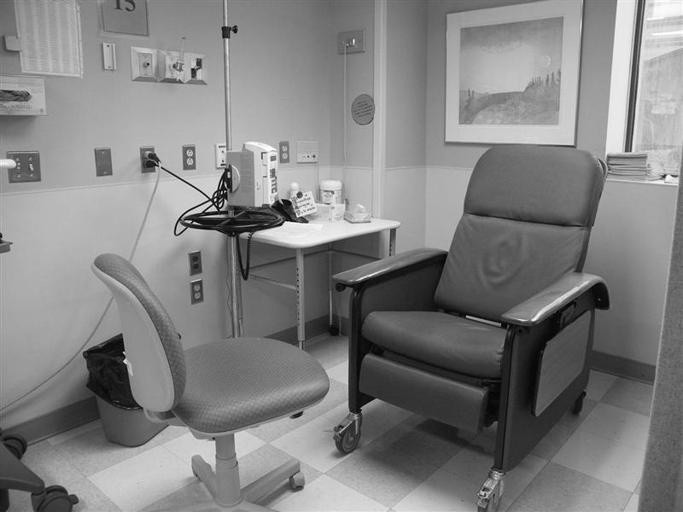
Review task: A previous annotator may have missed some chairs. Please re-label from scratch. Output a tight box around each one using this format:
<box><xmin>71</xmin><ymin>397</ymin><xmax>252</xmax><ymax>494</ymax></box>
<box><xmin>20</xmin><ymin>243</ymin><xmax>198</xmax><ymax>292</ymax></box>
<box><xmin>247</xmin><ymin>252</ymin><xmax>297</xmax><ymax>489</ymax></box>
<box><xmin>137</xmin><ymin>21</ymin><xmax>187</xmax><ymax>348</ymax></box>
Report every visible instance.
<box><xmin>91</xmin><ymin>253</ymin><xmax>330</xmax><ymax>512</ymax></box>
<box><xmin>329</xmin><ymin>147</ymin><xmax>611</xmax><ymax>512</ymax></box>
<box><xmin>0</xmin><ymin>426</ymin><xmax>79</xmax><ymax>511</ymax></box>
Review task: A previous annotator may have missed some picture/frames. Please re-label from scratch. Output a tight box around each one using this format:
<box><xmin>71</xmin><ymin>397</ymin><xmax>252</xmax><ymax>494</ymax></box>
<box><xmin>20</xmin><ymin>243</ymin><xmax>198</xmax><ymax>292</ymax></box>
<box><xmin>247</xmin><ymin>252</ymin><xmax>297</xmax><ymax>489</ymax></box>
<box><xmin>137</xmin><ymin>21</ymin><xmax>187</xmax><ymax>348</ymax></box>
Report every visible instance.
<box><xmin>444</xmin><ymin>0</ymin><xmax>585</xmax><ymax>149</ymax></box>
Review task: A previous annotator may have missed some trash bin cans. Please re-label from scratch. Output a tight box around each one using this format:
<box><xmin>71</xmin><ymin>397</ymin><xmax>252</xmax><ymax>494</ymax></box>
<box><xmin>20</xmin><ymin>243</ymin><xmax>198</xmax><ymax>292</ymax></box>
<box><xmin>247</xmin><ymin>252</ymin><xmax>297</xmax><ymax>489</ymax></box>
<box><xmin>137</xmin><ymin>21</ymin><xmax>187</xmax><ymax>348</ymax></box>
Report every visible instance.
<box><xmin>82</xmin><ymin>332</ymin><xmax>182</xmax><ymax>447</ymax></box>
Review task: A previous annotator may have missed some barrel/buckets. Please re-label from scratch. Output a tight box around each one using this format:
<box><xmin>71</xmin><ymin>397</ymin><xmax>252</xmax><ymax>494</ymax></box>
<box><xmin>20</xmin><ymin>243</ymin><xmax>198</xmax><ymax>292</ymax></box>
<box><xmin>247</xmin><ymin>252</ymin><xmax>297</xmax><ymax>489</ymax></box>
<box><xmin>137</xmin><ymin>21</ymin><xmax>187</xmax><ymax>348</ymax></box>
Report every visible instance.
<box><xmin>320</xmin><ymin>180</ymin><xmax>342</xmax><ymax>205</ymax></box>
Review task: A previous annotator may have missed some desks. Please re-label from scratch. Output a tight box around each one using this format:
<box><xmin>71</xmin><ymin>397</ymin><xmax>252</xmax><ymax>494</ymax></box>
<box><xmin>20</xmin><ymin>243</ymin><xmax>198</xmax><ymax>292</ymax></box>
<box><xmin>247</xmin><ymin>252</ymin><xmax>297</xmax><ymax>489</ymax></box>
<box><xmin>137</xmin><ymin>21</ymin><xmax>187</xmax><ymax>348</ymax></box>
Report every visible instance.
<box><xmin>227</xmin><ymin>204</ymin><xmax>400</xmax><ymax>351</ymax></box>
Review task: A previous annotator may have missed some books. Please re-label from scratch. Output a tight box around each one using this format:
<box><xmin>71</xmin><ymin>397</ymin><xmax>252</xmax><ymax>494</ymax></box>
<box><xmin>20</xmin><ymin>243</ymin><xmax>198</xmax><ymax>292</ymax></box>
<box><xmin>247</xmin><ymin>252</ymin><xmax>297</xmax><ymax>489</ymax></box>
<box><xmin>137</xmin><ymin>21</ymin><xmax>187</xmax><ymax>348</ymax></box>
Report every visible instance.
<box><xmin>606</xmin><ymin>151</ymin><xmax>649</xmax><ymax>181</ymax></box>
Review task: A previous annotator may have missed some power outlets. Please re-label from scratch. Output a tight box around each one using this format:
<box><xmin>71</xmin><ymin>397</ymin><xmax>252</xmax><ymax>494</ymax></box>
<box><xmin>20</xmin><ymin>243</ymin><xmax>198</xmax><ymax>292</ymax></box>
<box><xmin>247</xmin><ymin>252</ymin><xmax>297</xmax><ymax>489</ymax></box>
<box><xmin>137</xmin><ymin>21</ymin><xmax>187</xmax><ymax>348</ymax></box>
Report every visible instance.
<box><xmin>184</xmin><ymin>145</ymin><xmax>196</xmax><ymax>170</ymax></box>
<box><xmin>141</xmin><ymin>146</ymin><xmax>156</xmax><ymax>172</ymax></box>
